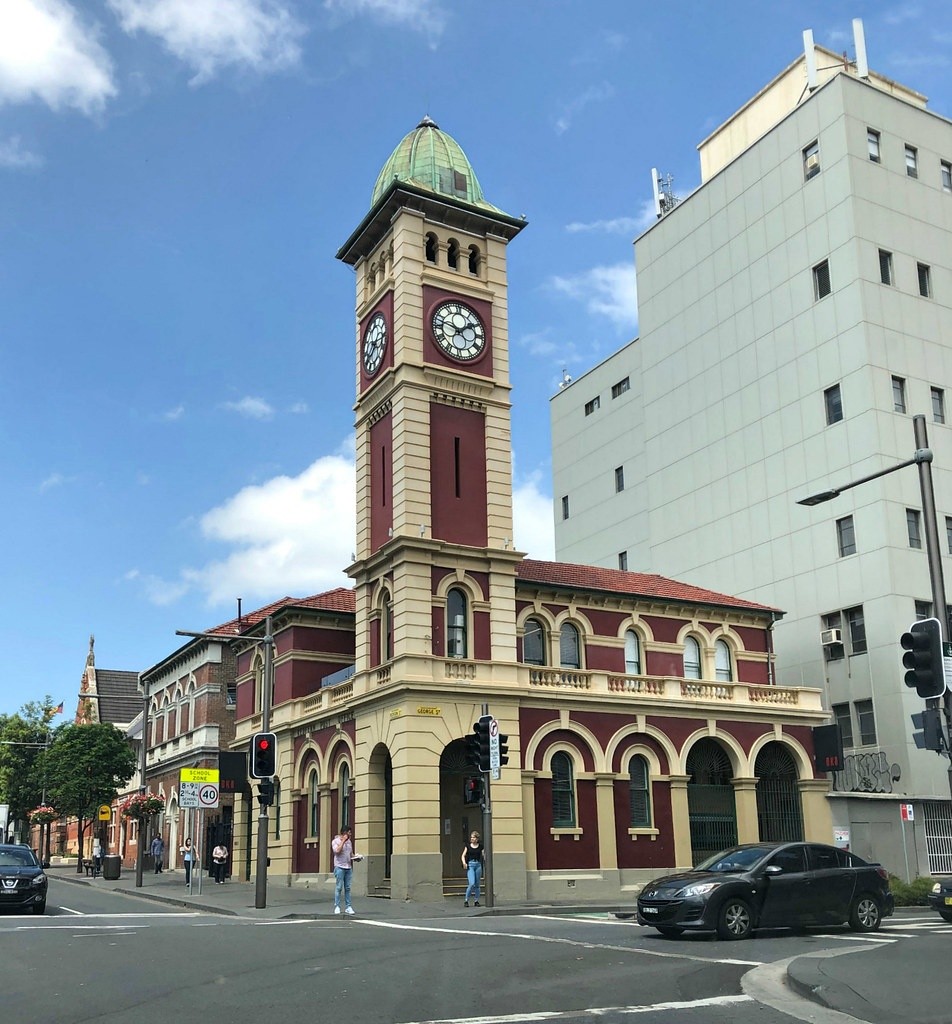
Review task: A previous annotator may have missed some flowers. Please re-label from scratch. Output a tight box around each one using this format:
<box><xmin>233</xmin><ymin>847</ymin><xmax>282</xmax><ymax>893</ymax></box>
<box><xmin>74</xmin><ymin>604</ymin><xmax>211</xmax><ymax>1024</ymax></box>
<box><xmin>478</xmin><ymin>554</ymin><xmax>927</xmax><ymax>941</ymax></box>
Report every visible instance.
<box><xmin>29</xmin><ymin>806</ymin><xmax>55</xmax><ymax>824</ymax></box>
<box><xmin>119</xmin><ymin>791</ymin><xmax>164</xmax><ymax>818</ymax></box>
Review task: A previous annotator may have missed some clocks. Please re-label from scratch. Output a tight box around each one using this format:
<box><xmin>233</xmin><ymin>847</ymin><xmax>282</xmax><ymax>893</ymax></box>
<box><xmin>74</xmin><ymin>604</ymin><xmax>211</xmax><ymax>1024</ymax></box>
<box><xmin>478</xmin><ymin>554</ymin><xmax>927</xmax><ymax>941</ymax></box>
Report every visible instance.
<box><xmin>428</xmin><ymin>296</ymin><xmax>489</xmax><ymax>365</ymax></box>
<box><xmin>359</xmin><ymin>311</ymin><xmax>387</xmax><ymax>379</ymax></box>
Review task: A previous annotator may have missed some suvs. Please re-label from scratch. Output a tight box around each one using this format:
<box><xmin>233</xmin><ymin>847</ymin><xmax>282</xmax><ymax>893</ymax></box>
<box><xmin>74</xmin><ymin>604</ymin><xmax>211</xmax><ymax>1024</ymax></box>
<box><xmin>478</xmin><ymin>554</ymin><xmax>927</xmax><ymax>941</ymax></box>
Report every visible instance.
<box><xmin>0</xmin><ymin>842</ymin><xmax>51</xmax><ymax>915</ymax></box>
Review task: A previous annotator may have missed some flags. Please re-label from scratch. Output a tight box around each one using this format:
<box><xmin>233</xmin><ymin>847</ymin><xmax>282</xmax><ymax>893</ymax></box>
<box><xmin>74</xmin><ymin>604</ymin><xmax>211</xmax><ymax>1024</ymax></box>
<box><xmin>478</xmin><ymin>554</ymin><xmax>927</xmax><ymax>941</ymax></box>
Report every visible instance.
<box><xmin>51</xmin><ymin>702</ymin><xmax>64</xmax><ymax>713</ymax></box>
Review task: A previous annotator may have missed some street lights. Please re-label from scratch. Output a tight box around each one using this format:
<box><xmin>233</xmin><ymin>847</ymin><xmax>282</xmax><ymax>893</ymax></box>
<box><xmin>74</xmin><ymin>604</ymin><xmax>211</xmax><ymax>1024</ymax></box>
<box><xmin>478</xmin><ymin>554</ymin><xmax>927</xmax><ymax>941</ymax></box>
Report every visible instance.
<box><xmin>2</xmin><ymin>733</ymin><xmax>48</xmax><ymax>864</ymax></box>
<box><xmin>175</xmin><ymin>616</ymin><xmax>274</xmax><ymax>908</ymax></box>
<box><xmin>795</xmin><ymin>414</ymin><xmax>952</xmax><ymax>798</ymax></box>
<box><xmin>78</xmin><ymin>681</ymin><xmax>151</xmax><ymax>887</ymax></box>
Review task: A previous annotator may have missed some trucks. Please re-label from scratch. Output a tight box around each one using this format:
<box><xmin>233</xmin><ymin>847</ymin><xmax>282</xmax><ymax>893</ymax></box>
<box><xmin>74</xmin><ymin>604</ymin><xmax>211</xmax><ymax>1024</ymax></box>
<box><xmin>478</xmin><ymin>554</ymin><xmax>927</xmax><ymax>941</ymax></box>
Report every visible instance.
<box><xmin>0</xmin><ymin>804</ymin><xmax>8</xmax><ymax>843</ymax></box>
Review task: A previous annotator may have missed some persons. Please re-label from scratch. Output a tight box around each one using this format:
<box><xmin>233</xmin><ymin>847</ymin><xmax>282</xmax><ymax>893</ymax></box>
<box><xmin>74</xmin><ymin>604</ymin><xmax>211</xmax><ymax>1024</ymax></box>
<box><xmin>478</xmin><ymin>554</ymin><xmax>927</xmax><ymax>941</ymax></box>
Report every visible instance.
<box><xmin>461</xmin><ymin>831</ymin><xmax>486</xmax><ymax>907</ymax></box>
<box><xmin>184</xmin><ymin>838</ymin><xmax>200</xmax><ymax>886</ymax></box>
<box><xmin>151</xmin><ymin>833</ymin><xmax>165</xmax><ymax>874</ymax></box>
<box><xmin>332</xmin><ymin>826</ymin><xmax>361</xmax><ymax>915</ymax></box>
<box><xmin>213</xmin><ymin>841</ymin><xmax>228</xmax><ymax>884</ymax></box>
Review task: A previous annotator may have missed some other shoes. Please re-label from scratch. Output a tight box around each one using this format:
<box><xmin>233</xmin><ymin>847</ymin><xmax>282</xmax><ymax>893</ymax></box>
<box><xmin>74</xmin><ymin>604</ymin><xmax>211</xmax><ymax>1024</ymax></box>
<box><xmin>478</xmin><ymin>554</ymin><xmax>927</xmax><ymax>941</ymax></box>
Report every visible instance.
<box><xmin>464</xmin><ymin>901</ymin><xmax>468</xmax><ymax>907</ymax></box>
<box><xmin>475</xmin><ymin>901</ymin><xmax>480</xmax><ymax>907</ymax></box>
<box><xmin>186</xmin><ymin>883</ymin><xmax>190</xmax><ymax>886</ymax></box>
<box><xmin>216</xmin><ymin>881</ymin><xmax>223</xmax><ymax>884</ymax></box>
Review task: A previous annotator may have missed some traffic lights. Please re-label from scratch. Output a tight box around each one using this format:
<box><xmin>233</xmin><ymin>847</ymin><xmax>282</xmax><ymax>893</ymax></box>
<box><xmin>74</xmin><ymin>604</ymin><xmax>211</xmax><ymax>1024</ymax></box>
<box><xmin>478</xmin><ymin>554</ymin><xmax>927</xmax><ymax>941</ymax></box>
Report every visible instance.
<box><xmin>464</xmin><ymin>776</ymin><xmax>483</xmax><ymax>804</ymax></box>
<box><xmin>911</xmin><ymin>709</ymin><xmax>943</xmax><ymax>750</ymax></box>
<box><xmin>900</xmin><ymin>617</ymin><xmax>945</xmax><ymax>699</ymax></box>
<box><xmin>253</xmin><ymin>733</ymin><xmax>277</xmax><ymax>778</ymax></box>
<box><xmin>473</xmin><ymin>714</ymin><xmax>494</xmax><ymax>772</ymax></box>
<box><xmin>258</xmin><ymin>782</ymin><xmax>274</xmax><ymax>806</ymax></box>
<box><xmin>499</xmin><ymin>734</ymin><xmax>509</xmax><ymax>766</ymax></box>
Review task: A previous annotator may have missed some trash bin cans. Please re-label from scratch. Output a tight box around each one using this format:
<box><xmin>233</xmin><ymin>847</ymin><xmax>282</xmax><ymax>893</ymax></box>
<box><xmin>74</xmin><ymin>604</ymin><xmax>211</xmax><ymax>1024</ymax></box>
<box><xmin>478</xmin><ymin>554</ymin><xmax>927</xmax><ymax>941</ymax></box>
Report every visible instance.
<box><xmin>104</xmin><ymin>855</ymin><xmax>121</xmax><ymax>880</ymax></box>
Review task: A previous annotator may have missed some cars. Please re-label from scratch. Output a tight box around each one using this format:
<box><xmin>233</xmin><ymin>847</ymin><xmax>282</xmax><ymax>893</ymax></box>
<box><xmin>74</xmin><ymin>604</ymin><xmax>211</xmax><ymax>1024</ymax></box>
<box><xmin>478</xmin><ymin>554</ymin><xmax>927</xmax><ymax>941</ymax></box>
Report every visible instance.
<box><xmin>927</xmin><ymin>876</ymin><xmax>952</xmax><ymax>923</ymax></box>
<box><xmin>637</xmin><ymin>841</ymin><xmax>895</xmax><ymax>941</ymax></box>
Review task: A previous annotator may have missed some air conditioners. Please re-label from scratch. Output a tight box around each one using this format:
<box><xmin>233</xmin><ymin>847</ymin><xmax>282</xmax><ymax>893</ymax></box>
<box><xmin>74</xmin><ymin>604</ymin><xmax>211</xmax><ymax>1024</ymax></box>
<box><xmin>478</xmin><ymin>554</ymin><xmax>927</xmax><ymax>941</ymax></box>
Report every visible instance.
<box><xmin>820</xmin><ymin>628</ymin><xmax>843</xmax><ymax>646</ymax></box>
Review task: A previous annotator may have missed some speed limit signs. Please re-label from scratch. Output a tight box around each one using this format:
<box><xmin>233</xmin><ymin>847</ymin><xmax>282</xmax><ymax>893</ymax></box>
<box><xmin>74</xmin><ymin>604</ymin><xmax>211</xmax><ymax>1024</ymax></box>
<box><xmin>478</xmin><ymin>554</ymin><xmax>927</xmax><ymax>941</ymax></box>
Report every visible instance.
<box><xmin>198</xmin><ymin>783</ymin><xmax>219</xmax><ymax>809</ymax></box>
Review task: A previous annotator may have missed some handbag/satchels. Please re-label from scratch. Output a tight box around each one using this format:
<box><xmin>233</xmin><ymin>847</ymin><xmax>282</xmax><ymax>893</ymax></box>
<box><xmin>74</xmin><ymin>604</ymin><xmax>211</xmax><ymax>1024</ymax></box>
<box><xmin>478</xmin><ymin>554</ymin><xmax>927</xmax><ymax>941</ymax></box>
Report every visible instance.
<box><xmin>218</xmin><ymin>857</ymin><xmax>225</xmax><ymax>862</ymax></box>
<box><xmin>462</xmin><ymin>846</ymin><xmax>469</xmax><ymax>870</ymax></box>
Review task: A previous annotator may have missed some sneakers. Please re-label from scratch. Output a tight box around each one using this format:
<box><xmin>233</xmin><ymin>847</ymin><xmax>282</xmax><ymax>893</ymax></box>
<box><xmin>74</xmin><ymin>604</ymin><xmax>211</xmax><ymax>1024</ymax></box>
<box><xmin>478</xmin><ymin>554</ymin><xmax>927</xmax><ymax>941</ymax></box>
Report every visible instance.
<box><xmin>345</xmin><ymin>906</ymin><xmax>355</xmax><ymax>915</ymax></box>
<box><xmin>334</xmin><ymin>905</ymin><xmax>340</xmax><ymax>914</ymax></box>
